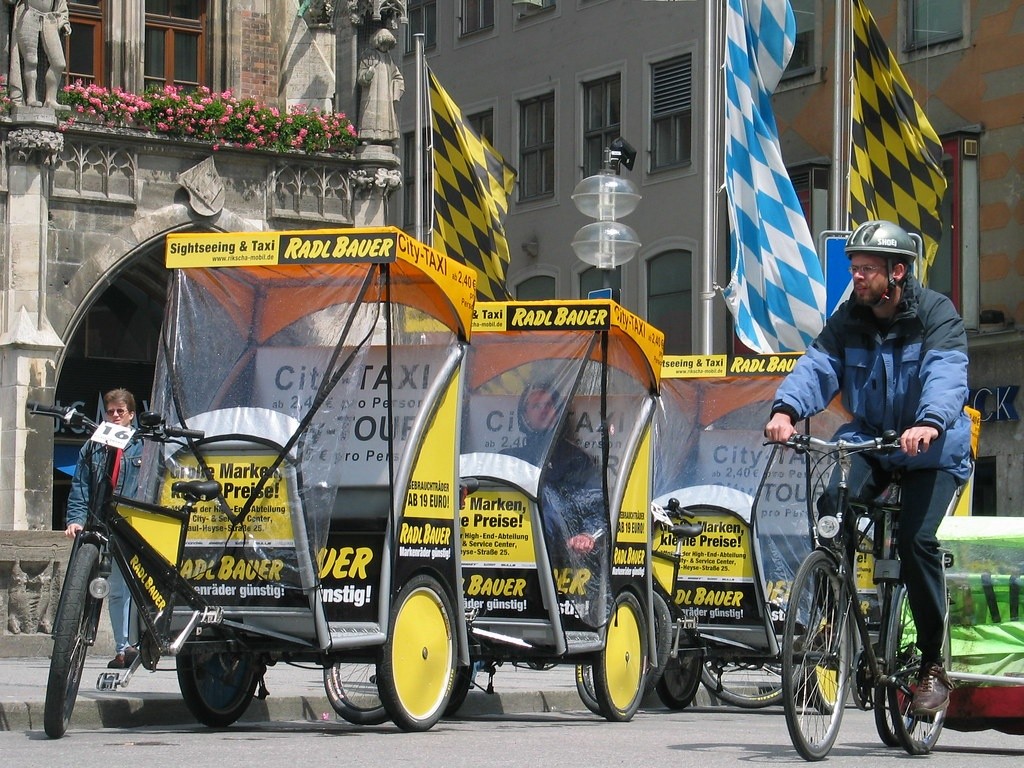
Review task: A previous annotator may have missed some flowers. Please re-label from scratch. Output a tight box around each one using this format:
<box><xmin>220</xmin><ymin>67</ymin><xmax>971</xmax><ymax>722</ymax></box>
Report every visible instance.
<box><xmin>0</xmin><ymin>76</ymin><xmax>18</xmax><ymax>118</ymax></box>
<box><xmin>58</xmin><ymin>80</ymin><xmax>366</xmax><ymax>159</ymax></box>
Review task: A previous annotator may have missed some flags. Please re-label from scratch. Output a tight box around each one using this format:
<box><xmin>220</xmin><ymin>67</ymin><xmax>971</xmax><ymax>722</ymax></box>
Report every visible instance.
<box><xmin>424</xmin><ymin>59</ymin><xmax>520</xmax><ymax>301</ymax></box>
<box><xmin>723</xmin><ymin>0</ymin><xmax>826</xmax><ymax>357</ymax></box>
<box><xmin>850</xmin><ymin>0</ymin><xmax>946</xmax><ymax>291</ymax></box>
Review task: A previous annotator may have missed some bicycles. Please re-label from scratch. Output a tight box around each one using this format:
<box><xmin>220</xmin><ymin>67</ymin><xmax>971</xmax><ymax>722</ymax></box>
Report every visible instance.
<box><xmin>760</xmin><ymin>429</ymin><xmax>958</xmax><ymax>762</ymax></box>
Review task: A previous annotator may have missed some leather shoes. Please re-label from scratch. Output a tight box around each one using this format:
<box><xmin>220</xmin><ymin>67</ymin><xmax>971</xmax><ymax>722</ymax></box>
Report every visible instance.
<box><xmin>912</xmin><ymin>662</ymin><xmax>953</xmax><ymax>711</ymax></box>
<box><xmin>796</xmin><ymin>621</ymin><xmax>841</xmax><ymax>667</ymax></box>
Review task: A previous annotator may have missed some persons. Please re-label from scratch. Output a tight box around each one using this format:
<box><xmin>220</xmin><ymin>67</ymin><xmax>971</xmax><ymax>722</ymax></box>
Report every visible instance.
<box><xmin>65</xmin><ymin>387</ymin><xmax>165</xmax><ymax>669</ymax></box>
<box><xmin>355</xmin><ymin>25</ymin><xmax>405</xmax><ymax>151</ymax></box>
<box><xmin>9</xmin><ymin>0</ymin><xmax>72</xmax><ymax>113</ymax></box>
<box><xmin>765</xmin><ymin>222</ymin><xmax>973</xmax><ymax>717</ymax></box>
<box><xmin>490</xmin><ymin>382</ymin><xmax>613</xmax><ymax>620</ymax></box>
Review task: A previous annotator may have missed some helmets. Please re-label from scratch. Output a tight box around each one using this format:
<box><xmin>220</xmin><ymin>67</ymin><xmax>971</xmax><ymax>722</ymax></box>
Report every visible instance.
<box><xmin>844</xmin><ymin>220</ymin><xmax>918</xmax><ymax>258</ymax></box>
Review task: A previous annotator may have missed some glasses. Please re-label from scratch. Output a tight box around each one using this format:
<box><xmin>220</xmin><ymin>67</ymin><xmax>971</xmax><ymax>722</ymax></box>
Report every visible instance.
<box><xmin>849</xmin><ymin>265</ymin><xmax>888</xmax><ymax>275</ymax></box>
<box><xmin>105</xmin><ymin>408</ymin><xmax>132</xmax><ymax>416</ymax></box>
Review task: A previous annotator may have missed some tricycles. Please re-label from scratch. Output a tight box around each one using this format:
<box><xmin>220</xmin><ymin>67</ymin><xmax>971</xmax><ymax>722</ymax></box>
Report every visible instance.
<box><xmin>571</xmin><ymin>351</ymin><xmax>889</xmax><ymax>716</ymax></box>
<box><xmin>24</xmin><ymin>224</ymin><xmax>482</xmax><ymax>743</ymax></box>
<box><xmin>321</xmin><ymin>296</ymin><xmax>667</xmax><ymax>734</ymax></box>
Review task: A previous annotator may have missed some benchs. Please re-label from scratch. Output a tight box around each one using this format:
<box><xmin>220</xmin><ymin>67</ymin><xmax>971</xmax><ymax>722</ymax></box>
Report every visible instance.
<box><xmin>311</xmin><ymin>484</ymin><xmax>390</xmax><ymax>537</ymax></box>
<box><xmin>757</xmin><ymin>528</ymin><xmax>812</xmax><ymax>576</ymax></box>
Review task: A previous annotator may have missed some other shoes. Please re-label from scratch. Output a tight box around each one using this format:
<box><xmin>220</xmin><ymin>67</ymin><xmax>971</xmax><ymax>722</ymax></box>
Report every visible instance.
<box><xmin>123</xmin><ymin>646</ymin><xmax>139</xmax><ymax>668</ymax></box>
<box><xmin>107</xmin><ymin>654</ymin><xmax>125</xmax><ymax>669</ymax></box>
